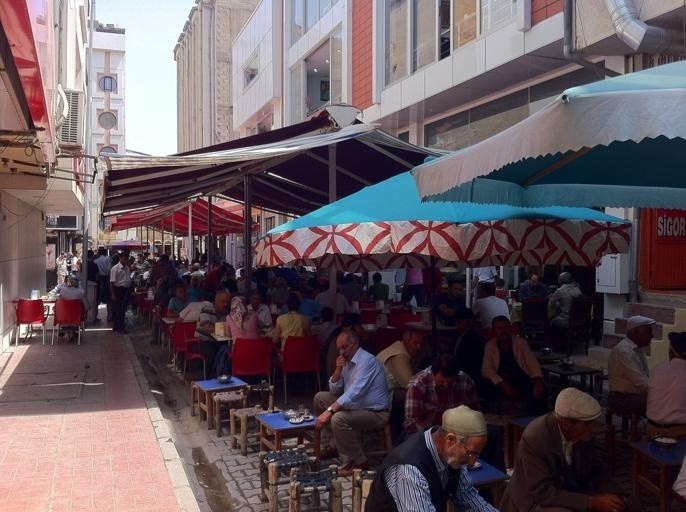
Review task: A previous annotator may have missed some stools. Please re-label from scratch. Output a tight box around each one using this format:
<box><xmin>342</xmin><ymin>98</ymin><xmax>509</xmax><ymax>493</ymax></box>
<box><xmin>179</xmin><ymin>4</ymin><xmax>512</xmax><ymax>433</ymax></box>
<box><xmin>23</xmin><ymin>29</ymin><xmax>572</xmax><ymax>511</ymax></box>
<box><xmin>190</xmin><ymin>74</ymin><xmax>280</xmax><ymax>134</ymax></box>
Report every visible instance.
<box><xmin>191</xmin><ymin>344</ymin><xmax>686</xmax><ymax>511</ymax></box>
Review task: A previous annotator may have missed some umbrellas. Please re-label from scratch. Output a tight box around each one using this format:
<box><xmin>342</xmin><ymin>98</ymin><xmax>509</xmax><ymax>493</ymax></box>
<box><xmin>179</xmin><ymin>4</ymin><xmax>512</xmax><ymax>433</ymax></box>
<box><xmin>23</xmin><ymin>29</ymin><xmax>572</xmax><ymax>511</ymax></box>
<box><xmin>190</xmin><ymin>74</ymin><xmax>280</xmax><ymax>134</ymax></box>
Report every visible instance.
<box><xmin>410</xmin><ymin>59</ymin><xmax>686</xmax><ymax>211</ymax></box>
<box><xmin>251</xmin><ymin>156</ymin><xmax>632</xmax><ymax>362</ymax></box>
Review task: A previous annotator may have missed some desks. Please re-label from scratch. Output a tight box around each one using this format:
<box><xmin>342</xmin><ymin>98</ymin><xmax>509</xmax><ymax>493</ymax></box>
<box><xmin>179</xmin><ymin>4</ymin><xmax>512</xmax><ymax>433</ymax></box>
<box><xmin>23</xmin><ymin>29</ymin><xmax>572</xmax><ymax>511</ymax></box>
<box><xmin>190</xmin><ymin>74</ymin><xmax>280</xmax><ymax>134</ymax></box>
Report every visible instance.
<box><xmin>9</xmin><ymin>296</ymin><xmax>58</xmax><ymax>342</ymax></box>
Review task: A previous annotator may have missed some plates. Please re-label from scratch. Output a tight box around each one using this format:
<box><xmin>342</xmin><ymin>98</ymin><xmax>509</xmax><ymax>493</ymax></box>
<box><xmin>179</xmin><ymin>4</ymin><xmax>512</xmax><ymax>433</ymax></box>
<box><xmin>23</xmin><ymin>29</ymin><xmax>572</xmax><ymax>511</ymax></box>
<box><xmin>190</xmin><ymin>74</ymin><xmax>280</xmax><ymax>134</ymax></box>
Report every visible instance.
<box><xmin>542</xmin><ymin>347</ymin><xmax>576</xmax><ymax>369</ymax></box>
<box><xmin>285</xmin><ymin>406</ymin><xmax>314</xmax><ymax>424</ymax></box>
<box><xmin>468</xmin><ymin>462</ymin><xmax>481</xmax><ymax>470</ymax></box>
<box><xmin>362</xmin><ymin>323</ymin><xmax>378</xmax><ymax>331</ymax></box>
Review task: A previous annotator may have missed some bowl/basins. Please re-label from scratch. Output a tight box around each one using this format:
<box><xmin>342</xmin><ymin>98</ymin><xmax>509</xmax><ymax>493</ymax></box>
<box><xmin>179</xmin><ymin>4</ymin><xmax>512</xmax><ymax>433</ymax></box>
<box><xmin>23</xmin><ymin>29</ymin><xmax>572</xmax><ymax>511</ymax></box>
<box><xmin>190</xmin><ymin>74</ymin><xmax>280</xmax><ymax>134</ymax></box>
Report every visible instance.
<box><xmin>218</xmin><ymin>373</ymin><xmax>232</xmax><ymax>383</ymax></box>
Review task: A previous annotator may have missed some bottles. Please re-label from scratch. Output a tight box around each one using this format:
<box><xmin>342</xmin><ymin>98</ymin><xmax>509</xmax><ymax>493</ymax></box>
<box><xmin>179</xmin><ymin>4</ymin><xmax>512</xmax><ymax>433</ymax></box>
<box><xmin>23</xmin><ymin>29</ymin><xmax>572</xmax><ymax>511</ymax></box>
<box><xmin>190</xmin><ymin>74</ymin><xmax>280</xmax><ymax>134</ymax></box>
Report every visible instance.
<box><xmin>148</xmin><ymin>291</ymin><xmax>153</xmax><ymax>299</ymax></box>
<box><xmin>31</xmin><ymin>290</ymin><xmax>41</xmax><ymax>300</ymax></box>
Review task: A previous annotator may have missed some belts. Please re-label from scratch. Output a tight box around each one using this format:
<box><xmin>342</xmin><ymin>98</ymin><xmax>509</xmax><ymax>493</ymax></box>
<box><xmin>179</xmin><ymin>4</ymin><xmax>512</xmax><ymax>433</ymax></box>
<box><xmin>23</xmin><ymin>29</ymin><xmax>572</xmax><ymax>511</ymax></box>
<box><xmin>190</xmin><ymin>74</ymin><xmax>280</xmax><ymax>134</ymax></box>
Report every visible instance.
<box><xmin>647</xmin><ymin>417</ymin><xmax>686</xmax><ymax>429</ymax></box>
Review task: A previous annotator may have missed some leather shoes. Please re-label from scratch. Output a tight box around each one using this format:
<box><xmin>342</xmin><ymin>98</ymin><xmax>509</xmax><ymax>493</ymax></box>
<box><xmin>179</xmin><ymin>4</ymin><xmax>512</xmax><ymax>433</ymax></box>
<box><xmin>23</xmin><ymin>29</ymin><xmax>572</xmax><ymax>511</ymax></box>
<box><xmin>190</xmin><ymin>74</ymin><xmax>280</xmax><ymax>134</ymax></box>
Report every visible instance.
<box><xmin>318</xmin><ymin>444</ymin><xmax>339</xmax><ymax>460</ymax></box>
<box><xmin>336</xmin><ymin>459</ymin><xmax>369</xmax><ymax>478</ymax></box>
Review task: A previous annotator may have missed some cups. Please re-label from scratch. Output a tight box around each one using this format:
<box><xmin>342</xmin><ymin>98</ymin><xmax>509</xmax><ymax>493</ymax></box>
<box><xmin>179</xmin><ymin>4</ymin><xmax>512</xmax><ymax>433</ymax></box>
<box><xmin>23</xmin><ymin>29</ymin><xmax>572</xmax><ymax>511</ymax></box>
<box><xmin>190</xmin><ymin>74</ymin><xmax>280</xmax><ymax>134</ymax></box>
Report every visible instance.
<box><xmin>656</xmin><ymin>437</ymin><xmax>678</xmax><ymax>446</ymax></box>
<box><xmin>42</xmin><ymin>294</ymin><xmax>59</xmax><ymax>300</ymax></box>
<box><xmin>352</xmin><ymin>301</ymin><xmax>359</xmax><ymax>310</ymax></box>
<box><xmin>376</xmin><ymin>300</ymin><xmax>384</xmax><ymax>310</ymax></box>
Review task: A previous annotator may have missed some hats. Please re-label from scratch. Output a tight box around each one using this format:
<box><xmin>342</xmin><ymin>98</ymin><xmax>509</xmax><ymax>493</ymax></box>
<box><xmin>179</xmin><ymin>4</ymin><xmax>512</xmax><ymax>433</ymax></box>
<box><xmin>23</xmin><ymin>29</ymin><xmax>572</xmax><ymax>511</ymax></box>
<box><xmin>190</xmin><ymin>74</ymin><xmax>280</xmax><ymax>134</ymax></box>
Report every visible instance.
<box><xmin>667</xmin><ymin>331</ymin><xmax>686</xmax><ymax>359</ymax></box>
<box><xmin>440</xmin><ymin>403</ymin><xmax>489</xmax><ymax>438</ymax></box>
<box><xmin>625</xmin><ymin>314</ymin><xmax>657</xmax><ymax>331</ymax></box>
<box><xmin>553</xmin><ymin>386</ymin><xmax>605</xmax><ymax>422</ymax></box>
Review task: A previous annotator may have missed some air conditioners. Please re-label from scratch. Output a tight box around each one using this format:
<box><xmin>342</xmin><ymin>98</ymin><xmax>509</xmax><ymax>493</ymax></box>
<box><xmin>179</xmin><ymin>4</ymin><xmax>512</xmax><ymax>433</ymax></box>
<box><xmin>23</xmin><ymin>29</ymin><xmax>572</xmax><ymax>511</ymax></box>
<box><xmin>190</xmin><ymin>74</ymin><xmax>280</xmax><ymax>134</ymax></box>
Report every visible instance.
<box><xmin>58</xmin><ymin>89</ymin><xmax>86</xmax><ymax>149</ymax></box>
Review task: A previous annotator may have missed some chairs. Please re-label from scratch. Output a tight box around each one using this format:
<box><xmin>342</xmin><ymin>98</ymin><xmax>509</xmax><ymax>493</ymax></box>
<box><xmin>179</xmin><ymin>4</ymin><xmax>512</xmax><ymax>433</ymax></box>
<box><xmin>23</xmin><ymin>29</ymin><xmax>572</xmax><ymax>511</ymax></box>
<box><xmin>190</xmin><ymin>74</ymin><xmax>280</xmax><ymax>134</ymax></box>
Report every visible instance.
<box><xmin>121</xmin><ymin>268</ymin><xmax>398</xmax><ymax>405</ymax></box>
<box><xmin>360</xmin><ymin>295</ymin><xmax>595</xmax><ymax>372</ymax></box>
<box><xmin>50</xmin><ymin>299</ymin><xmax>86</xmax><ymax>345</ymax></box>
<box><xmin>14</xmin><ymin>297</ymin><xmax>49</xmax><ymax>346</ymax></box>
